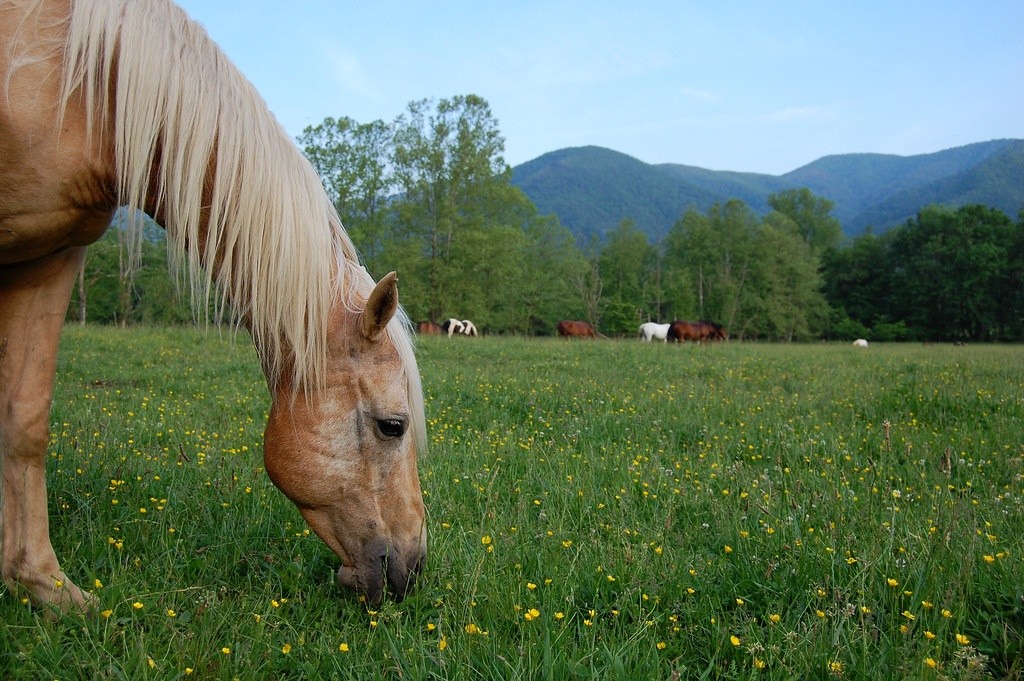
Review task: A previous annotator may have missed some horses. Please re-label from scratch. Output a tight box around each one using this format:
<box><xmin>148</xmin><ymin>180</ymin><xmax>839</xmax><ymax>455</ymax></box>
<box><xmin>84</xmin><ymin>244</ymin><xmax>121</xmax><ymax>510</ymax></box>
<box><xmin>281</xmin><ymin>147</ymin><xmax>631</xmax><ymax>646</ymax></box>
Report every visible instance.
<box><xmin>411</xmin><ymin>314</ymin><xmax>728</xmax><ymax>347</ymax></box>
<box><xmin>0</xmin><ymin>1</ymin><xmax>430</xmax><ymax>622</ymax></box>
<box><xmin>853</xmin><ymin>338</ymin><xmax>869</xmax><ymax>347</ymax></box>
